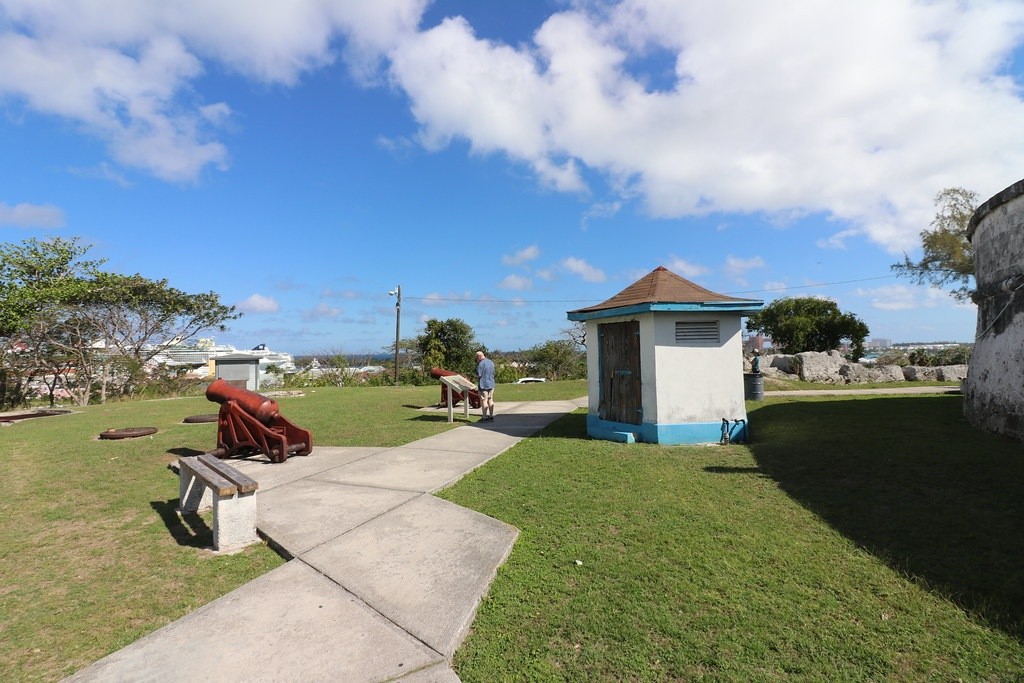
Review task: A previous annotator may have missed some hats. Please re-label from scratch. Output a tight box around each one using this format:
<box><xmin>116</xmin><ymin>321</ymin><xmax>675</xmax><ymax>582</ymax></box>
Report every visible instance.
<box><xmin>474</xmin><ymin>351</ymin><xmax>484</xmax><ymax>356</ymax></box>
<box><xmin>750</xmin><ymin>349</ymin><xmax>759</xmax><ymax>353</ymax></box>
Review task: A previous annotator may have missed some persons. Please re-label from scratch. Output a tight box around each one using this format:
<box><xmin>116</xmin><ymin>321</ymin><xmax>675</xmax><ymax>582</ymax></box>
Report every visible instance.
<box><xmin>749</xmin><ymin>348</ymin><xmax>760</xmax><ymax>373</ymax></box>
<box><xmin>473</xmin><ymin>351</ymin><xmax>496</xmax><ymax>423</ymax></box>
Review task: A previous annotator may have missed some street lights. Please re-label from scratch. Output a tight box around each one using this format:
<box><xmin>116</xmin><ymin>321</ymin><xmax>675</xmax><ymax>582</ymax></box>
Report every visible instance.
<box><xmin>388</xmin><ymin>288</ymin><xmax>401</xmax><ymax>382</ymax></box>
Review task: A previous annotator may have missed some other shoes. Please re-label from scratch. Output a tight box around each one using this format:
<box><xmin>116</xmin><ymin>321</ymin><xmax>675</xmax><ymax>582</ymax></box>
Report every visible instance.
<box><xmin>488</xmin><ymin>417</ymin><xmax>493</xmax><ymax>422</ymax></box>
<box><xmin>478</xmin><ymin>418</ymin><xmax>488</xmax><ymax>423</ymax></box>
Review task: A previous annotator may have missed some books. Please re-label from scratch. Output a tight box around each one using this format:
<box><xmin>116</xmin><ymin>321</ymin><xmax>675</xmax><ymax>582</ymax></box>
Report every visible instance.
<box><xmin>744</xmin><ymin>353</ymin><xmax>750</xmax><ymax>361</ymax></box>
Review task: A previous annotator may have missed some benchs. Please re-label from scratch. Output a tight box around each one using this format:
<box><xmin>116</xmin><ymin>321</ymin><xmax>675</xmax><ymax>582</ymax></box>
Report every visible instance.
<box><xmin>178</xmin><ymin>454</ymin><xmax>259</xmax><ymax>551</ymax></box>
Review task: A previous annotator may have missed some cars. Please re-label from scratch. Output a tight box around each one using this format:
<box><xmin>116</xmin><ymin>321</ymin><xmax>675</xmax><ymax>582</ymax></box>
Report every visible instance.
<box><xmin>513</xmin><ymin>378</ymin><xmax>546</xmax><ymax>384</ymax></box>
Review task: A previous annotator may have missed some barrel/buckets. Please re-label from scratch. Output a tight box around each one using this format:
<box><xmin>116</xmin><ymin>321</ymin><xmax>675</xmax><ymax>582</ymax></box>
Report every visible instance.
<box><xmin>744</xmin><ymin>374</ymin><xmax>763</xmax><ymax>402</ymax></box>
